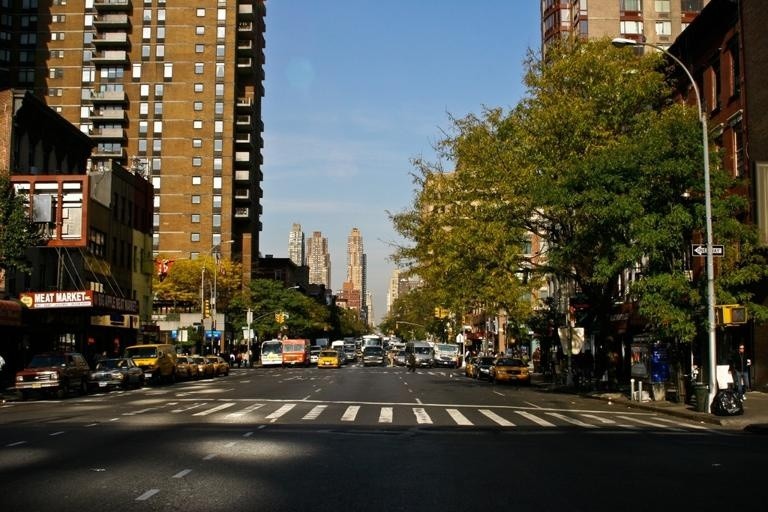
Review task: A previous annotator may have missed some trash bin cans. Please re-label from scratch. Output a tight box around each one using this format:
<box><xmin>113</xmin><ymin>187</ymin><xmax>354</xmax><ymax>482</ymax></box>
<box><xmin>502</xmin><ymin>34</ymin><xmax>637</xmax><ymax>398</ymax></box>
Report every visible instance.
<box><xmin>695</xmin><ymin>386</ymin><xmax>709</xmax><ymax>411</ymax></box>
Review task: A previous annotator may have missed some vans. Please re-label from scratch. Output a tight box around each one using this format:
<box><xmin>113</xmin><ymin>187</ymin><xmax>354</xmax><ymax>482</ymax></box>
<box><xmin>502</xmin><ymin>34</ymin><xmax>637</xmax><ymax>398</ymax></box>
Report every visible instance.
<box><xmin>124</xmin><ymin>343</ymin><xmax>179</xmax><ymax>387</ymax></box>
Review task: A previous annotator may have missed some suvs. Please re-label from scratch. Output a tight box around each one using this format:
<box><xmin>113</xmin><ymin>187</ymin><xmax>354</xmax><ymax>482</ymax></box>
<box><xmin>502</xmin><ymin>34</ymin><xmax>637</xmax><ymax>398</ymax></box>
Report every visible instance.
<box><xmin>14</xmin><ymin>352</ymin><xmax>93</xmax><ymax>401</ymax></box>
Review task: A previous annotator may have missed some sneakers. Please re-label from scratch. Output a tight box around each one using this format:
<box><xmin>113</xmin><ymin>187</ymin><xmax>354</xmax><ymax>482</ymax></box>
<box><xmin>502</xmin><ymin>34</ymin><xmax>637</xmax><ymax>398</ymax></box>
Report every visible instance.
<box><xmin>739</xmin><ymin>398</ymin><xmax>744</xmax><ymax>404</ymax></box>
<box><xmin>743</xmin><ymin>394</ymin><xmax>748</xmax><ymax>401</ymax></box>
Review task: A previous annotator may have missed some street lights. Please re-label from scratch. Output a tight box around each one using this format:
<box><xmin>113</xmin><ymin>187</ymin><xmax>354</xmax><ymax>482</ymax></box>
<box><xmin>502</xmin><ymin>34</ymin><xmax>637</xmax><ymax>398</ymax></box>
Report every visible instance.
<box><xmin>611</xmin><ymin>36</ymin><xmax>720</xmax><ymax>417</ymax></box>
<box><xmin>288</xmin><ymin>286</ymin><xmax>300</xmax><ymax>290</ymax></box>
<box><xmin>201</xmin><ymin>239</ymin><xmax>235</xmax><ymax>323</ymax></box>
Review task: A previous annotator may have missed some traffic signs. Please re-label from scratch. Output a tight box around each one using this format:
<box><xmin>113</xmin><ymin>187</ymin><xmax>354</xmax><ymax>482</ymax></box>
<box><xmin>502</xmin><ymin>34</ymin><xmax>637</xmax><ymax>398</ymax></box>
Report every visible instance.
<box><xmin>280</xmin><ymin>310</ymin><xmax>286</xmax><ymax>324</ymax></box>
<box><xmin>691</xmin><ymin>244</ymin><xmax>725</xmax><ymax>257</ymax></box>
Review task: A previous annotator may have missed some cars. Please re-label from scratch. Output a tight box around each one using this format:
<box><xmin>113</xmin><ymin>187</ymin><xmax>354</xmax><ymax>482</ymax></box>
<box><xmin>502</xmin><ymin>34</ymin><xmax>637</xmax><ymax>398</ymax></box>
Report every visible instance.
<box><xmin>174</xmin><ymin>354</ymin><xmax>230</xmax><ymax>380</ymax></box>
<box><xmin>466</xmin><ymin>356</ymin><xmax>531</xmax><ymax>387</ymax></box>
<box><xmin>85</xmin><ymin>357</ymin><xmax>144</xmax><ymax>394</ymax></box>
<box><xmin>259</xmin><ymin>331</ymin><xmax>462</xmax><ymax>370</ymax></box>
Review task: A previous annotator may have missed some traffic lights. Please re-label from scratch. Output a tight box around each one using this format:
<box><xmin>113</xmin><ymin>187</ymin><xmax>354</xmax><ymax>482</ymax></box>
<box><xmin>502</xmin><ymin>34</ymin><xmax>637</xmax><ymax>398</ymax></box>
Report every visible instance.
<box><xmin>204</xmin><ymin>299</ymin><xmax>210</xmax><ymax>319</ymax></box>
<box><xmin>275</xmin><ymin>312</ymin><xmax>280</xmax><ymax>324</ymax></box>
<box><xmin>731</xmin><ymin>307</ymin><xmax>746</xmax><ymax>323</ymax></box>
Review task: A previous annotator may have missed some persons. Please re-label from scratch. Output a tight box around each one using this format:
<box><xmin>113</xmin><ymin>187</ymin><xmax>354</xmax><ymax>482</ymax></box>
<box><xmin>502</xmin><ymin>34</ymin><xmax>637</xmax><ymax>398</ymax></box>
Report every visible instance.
<box><xmin>214</xmin><ymin>349</ymin><xmax>253</xmax><ymax>369</ymax></box>
<box><xmin>408</xmin><ymin>353</ymin><xmax>416</xmax><ymax>372</ymax></box>
<box><xmin>730</xmin><ymin>345</ymin><xmax>751</xmax><ymax>403</ymax></box>
